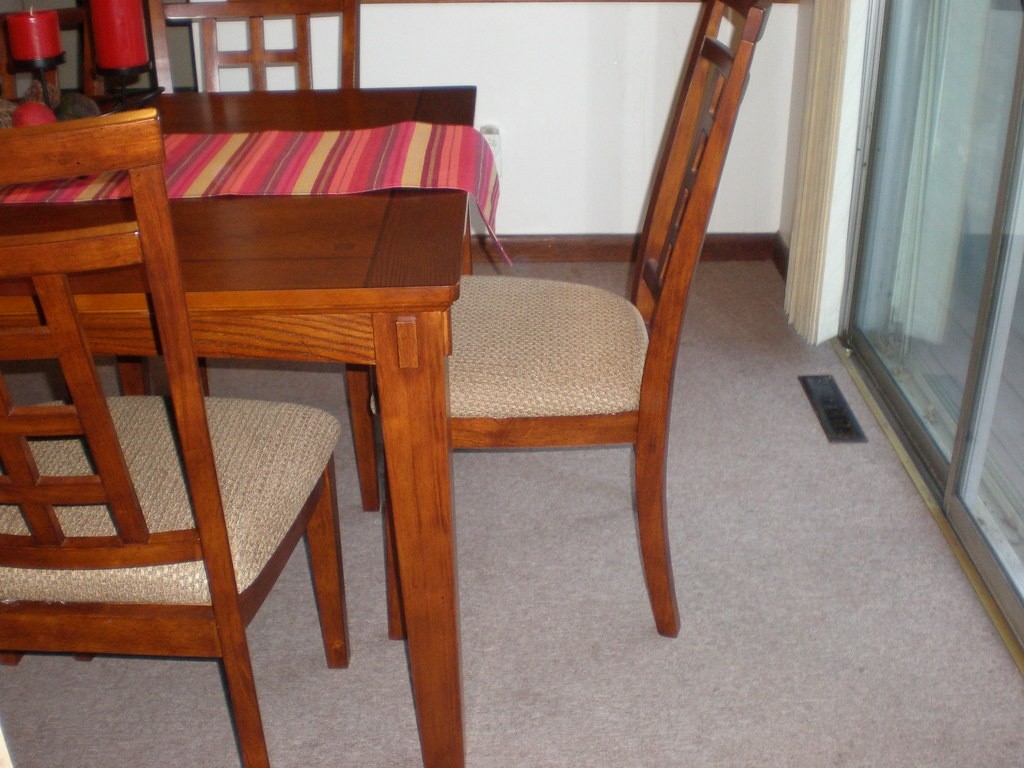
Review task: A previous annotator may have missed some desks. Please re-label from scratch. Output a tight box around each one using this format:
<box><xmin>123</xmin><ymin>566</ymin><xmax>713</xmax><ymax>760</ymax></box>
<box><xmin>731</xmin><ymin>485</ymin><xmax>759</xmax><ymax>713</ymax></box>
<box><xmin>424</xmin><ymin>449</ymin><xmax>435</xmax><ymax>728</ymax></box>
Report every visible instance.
<box><xmin>0</xmin><ymin>85</ymin><xmax>475</xmax><ymax>768</ymax></box>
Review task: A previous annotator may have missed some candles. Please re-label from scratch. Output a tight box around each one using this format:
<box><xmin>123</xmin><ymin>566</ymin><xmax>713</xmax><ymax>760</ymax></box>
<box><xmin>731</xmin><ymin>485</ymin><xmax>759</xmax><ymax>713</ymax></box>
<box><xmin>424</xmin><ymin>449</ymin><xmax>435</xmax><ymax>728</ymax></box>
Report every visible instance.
<box><xmin>7</xmin><ymin>7</ymin><xmax>62</xmax><ymax>60</ymax></box>
<box><xmin>90</xmin><ymin>0</ymin><xmax>150</xmax><ymax>71</ymax></box>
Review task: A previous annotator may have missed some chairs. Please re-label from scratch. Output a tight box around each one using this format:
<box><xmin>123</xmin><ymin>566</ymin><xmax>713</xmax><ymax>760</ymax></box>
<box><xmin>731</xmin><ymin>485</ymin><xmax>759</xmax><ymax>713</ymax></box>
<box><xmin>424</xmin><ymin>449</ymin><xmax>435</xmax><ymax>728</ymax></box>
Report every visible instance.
<box><xmin>371</xmin><ymin>1</ymin><xmax>772</xmax><ymax>640</ymax></box>
<box><xmin>0</xmin><ymin>108</ymin><xmax>350</xmax><ymax>767</ymax></box>
<box><xmin>114</xmin><ymin>1</ymin><xmax>383</xmax><ymax>512</ymax></box>
